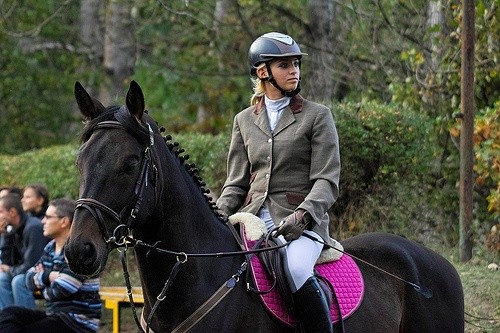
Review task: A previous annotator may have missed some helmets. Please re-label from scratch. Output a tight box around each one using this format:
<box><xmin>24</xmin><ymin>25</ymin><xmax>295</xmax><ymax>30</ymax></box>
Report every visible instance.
<box><xmin>247</xmin><ymin>32</ymin><xmax>309</xmax><ymax>75</ymax></box>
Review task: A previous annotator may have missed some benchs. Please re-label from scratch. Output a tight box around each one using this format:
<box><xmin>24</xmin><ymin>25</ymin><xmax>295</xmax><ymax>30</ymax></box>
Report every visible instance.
<box><xmin>34</xmin><ymin>287</ymin><xmax>144</xmax><ymax>333</ymax></box>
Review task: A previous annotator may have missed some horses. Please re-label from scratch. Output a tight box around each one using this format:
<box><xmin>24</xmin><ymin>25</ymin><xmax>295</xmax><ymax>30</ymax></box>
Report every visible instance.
<box><xmin>66</xmin><ymin>80</ymin><xmax>465</xmax><ymax>333</ymax></box>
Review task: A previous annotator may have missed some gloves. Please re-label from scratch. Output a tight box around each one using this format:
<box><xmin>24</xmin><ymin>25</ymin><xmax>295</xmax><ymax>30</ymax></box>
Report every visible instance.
<box><xmin>272</xmin><ymin>209</ymin><xmax>313</xmax><ymax>241</ymax></box>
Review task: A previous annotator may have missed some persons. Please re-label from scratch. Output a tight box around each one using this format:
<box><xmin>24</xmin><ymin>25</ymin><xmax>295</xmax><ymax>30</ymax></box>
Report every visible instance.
<box><xmin>0</xmin><ymin>199</ymin><xmax>102</xmax><ymax>333</ymax></box>
<box><xmin>215</xmin><ymin>32</ymin><xmax>340</xmax><ymax>333</ymax></box>
<box><xmin>0</xmin><ymin>185</ymin><xmax>49</xmax><ymax>309</ymax></box>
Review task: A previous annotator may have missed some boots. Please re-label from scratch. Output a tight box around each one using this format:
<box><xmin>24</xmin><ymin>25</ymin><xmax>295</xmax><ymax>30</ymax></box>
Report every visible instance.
<box><xmin>292</xmin><ymin>276</ymin><xmax>334</xmax><ymax>333</ymax></box>
